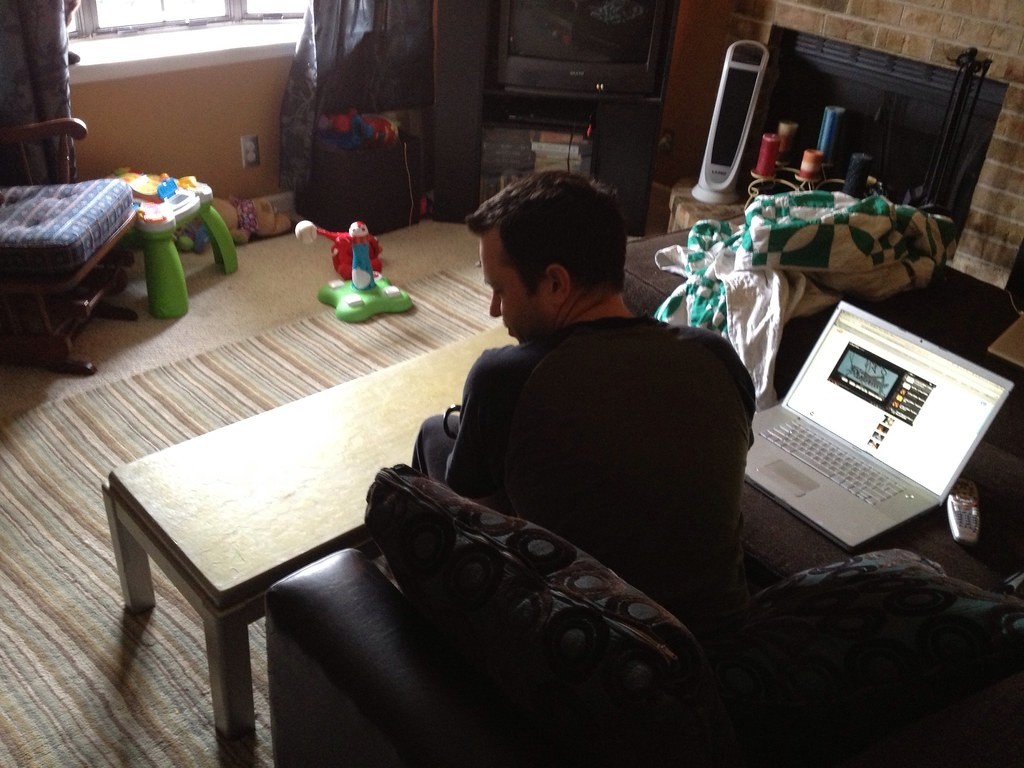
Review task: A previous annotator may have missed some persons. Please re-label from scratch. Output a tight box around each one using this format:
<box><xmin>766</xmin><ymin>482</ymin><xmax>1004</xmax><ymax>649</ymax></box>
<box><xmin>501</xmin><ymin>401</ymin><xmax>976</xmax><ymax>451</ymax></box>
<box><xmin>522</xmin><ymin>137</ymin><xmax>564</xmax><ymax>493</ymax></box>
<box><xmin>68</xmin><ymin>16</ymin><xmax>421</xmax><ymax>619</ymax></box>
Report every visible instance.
<box><xmin>412</xmin><ymin>169</ymin><xmax>756</xmax><ymax>638</ymax></box>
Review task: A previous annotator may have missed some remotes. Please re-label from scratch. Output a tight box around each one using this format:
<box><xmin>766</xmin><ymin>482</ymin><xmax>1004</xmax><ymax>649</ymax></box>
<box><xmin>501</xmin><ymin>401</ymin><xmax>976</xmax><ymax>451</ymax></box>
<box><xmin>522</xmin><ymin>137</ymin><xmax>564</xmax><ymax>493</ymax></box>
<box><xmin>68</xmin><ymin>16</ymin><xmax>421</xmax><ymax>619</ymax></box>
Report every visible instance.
<box><xmin>946</xmin><ymin>478</ymin><xmax>980</xmax><ymax>545</ymax></box>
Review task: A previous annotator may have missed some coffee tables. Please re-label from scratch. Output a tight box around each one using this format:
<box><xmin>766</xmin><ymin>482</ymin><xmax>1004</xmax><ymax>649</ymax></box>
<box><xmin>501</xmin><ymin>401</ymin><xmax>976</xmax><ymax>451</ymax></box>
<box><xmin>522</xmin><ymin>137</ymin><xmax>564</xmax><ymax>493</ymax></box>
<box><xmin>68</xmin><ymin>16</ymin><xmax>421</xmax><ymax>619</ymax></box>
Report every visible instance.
<box><xmin>100</xmin><ymin>324</ymin><xmax>520</xmax><ymax>742</ymax></box>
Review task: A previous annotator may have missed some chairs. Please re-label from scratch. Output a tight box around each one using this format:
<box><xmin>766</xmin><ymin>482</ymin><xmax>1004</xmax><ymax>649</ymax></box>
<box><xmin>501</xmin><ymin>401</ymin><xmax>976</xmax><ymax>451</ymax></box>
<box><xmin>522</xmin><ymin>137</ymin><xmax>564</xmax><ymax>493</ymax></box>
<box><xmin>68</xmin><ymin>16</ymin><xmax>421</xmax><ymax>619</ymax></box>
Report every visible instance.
<box><xmin>0</xmin><ymin>118</ymin><xmax>139</xmax><ymax>375</ymax></box>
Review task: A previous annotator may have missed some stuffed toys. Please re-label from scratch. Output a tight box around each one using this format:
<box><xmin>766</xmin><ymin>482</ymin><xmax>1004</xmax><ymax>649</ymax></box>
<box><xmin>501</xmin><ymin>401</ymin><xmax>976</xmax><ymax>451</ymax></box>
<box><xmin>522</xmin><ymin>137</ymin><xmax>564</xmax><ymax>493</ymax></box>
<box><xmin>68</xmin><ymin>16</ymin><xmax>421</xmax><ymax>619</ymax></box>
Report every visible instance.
<box><xmin>214</xmin><ymin>197</ymin><xmax>292</xmax><ymax>245</ymax></box>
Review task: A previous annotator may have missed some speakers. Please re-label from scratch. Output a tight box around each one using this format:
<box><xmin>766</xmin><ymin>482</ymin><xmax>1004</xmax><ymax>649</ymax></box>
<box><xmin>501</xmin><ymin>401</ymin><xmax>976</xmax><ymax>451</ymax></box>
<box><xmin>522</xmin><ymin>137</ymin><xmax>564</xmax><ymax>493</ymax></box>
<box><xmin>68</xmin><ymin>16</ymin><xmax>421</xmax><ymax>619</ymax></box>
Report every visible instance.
<box><xmin>692</xmin><ymin>40</ymin><xmax>769</xmax><ymax>203</ymax></box>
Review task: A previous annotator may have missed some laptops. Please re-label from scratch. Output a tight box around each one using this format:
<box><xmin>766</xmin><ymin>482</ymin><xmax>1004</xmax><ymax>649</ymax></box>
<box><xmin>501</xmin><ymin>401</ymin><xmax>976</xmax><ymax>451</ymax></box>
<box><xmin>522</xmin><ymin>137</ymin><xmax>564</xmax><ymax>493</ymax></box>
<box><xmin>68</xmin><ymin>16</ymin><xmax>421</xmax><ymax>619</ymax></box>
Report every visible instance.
<box><xmin>744</xmin><ymin>298</ymin><xmax>1014</xmax><ymax>548</ymax></box>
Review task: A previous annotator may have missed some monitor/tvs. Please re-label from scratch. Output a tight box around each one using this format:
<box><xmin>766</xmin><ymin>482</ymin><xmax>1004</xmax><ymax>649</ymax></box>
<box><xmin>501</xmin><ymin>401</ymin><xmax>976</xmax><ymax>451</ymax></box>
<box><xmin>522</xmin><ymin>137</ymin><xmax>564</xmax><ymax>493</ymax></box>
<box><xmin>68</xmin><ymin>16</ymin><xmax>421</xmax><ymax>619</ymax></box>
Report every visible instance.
<box><xmin>496</xmin><ymin>0</ymin><xmax>668</xmax><ymax>95</ymax></box>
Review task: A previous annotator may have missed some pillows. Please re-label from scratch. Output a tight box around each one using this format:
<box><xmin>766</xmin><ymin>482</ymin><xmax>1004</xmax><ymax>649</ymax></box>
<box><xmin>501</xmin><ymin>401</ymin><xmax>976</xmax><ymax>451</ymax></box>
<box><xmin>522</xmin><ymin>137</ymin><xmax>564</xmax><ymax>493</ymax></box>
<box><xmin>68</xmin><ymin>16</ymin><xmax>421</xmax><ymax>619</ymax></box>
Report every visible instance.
<box><xmin>696</xmin><ymin>548</ymin><xmax>1024</xmax><ymax>768</ymax></box>
<box><xmin>364</xmin><ymin>463</ymin><xmax>743</xmax><ymax>768</ymax></box>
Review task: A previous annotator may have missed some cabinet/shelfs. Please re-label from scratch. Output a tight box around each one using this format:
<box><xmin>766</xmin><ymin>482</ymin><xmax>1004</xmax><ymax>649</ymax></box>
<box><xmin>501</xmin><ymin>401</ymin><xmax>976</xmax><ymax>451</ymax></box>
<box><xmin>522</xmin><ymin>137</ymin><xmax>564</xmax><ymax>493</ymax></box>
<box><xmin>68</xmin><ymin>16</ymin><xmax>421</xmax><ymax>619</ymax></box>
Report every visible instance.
<box><xmin>428</xmin><ymin>0</ymin><xmax>680</xmax><ymax>238</ymax></box>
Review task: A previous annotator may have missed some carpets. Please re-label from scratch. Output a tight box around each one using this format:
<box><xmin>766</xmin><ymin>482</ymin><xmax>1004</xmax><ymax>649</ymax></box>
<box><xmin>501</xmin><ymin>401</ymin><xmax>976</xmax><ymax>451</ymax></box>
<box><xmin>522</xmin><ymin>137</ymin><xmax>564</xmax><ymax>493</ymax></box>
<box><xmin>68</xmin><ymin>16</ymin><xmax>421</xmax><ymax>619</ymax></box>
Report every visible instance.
<box><xmin>0</xmin><ymin>259</ymin><xmax>513</xmax><ymax>768</ymax></box>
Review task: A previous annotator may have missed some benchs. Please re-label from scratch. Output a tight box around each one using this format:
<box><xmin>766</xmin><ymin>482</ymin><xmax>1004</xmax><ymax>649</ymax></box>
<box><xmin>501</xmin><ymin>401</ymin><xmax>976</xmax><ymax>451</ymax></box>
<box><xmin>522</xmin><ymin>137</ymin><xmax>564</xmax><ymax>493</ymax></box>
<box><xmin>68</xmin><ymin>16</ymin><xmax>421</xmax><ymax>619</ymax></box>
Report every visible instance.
<box><xmin>621</xmin><ymin>213</ymin><xmax>1024</xmax><ymax>457</ymax></box>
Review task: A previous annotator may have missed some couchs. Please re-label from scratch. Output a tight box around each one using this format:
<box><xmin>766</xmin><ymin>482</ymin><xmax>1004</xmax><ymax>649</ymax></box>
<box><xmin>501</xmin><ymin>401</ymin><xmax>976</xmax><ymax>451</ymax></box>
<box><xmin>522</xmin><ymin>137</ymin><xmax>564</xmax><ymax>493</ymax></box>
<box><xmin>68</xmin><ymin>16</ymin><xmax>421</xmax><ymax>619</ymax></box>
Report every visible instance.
<box><xmin>262</xmin><ymin>402</ymin><xmax>1024</xmax><ymax>768</ymax></box>
